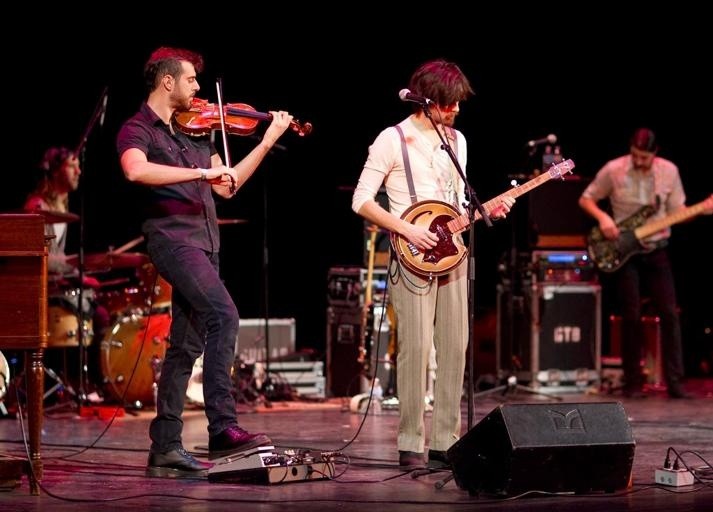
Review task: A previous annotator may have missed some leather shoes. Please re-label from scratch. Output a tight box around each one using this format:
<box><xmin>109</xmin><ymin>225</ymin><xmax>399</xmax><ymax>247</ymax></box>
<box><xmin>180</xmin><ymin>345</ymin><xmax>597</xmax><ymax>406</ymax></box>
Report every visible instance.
<box><xmin>208</xmin><ymin>425</ymin><xmax>271</xmax><ymax>461</ymax></box>
<box><xmin>146</xmin><ymin>448</ymin><xmax>213</xmax><ymax>476</ymax></box>
<box><xmin>399</xmin><ymin>452</ymin><xmax>427</xmax><ymax>470</ymax></box>
<box><xmin>428</xmin><ymin>450</ymin><xmax>451</xmax><ymax>467</ymax></box>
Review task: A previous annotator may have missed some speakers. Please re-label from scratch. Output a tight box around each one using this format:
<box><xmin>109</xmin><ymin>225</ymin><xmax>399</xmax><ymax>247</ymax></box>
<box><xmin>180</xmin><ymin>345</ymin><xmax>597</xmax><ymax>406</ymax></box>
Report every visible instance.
<box><xmin>447</xmin><ymin>401</ymin><xmax>635</xmax><ymax>498</ymax></box>
<box><xmin>610</xmin><ymin>316</ymin><xmax>663</xmax><ymax>386</ymax></box>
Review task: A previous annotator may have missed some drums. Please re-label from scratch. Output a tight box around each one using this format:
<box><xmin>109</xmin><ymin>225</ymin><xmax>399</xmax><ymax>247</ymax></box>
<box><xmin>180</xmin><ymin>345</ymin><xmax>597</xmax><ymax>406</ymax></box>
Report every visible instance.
<box><xmin>99</xmin><ymin>302</ymin><xmax>235</xmax><ymax>408</ymax></box>
<box><xmin>49</xmin><ymin>296</ymin><xmax>94</xmax><ymax>346</ymax></box>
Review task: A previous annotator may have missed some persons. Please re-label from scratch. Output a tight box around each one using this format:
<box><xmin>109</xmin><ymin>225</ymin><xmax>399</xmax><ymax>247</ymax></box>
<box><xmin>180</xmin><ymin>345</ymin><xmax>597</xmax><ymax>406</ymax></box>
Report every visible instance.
<box><xmin>349</xmin><ymin>58</ymin><xmax>517</xmax><ymax>472</ymax></box>
<box><xmin>114</xmin><ymin>43</ymin><xmax>295</xmax><ymax>476</ymax></box>
<box><xmin>575</xmin><ymin>125</ymin><xmax>713</xmax><ymax>401</ymax></box>
<box><xmin>24</xmin><ymin>140</ymin><xmax>103</xmax><ymax>386</ymax></box>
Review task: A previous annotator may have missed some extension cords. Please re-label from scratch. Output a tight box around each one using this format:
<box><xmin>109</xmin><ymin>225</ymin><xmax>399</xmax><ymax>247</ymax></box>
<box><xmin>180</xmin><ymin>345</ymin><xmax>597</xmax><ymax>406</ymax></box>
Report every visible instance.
<box><xmin>655</xmin><ymin>465</ymin><xmax>696</xmax><ymax>487</ymax></box>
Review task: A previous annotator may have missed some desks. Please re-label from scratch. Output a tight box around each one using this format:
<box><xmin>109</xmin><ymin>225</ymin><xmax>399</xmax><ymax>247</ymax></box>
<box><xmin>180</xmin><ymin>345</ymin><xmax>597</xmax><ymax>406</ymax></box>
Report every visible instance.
<box><xmin>0</xmin><ymin>213</ymin><xmax>56</xmax><ymax>495</ymax></box>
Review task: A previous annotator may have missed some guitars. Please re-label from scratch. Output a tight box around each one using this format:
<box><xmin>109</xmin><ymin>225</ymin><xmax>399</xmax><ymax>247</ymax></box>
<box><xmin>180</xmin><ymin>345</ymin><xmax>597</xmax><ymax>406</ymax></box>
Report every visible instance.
<box><xmin>584</xmin><ymin>194</ymin><xmax>712</xmax><ymax>272</ymax></box>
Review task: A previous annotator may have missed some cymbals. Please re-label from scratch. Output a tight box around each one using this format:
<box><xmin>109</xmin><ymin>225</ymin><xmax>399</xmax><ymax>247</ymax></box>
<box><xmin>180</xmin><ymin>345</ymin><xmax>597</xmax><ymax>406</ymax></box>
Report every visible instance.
<box><xmin>217</xmin><ymin>219</ymin><xmax>247</xmax><ymax>224</ymax></box>
<box><xmin>66</xmin><ymin>253</ymin><xmax>149</xmax><ymax>272</ymax></box>
<box><xmin>17</xmin><ymin>210</ymin><xmax>80</xmax><ymax>223</ymax></box>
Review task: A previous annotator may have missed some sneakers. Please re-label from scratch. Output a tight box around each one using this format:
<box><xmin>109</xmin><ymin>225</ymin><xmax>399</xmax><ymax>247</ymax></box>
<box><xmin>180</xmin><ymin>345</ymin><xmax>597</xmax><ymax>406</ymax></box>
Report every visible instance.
<box><xmin>669</xmin><ymin>384</ymin><xmax>697</xmax><ymax>399</ymax></box>
<box><xmin>624</xmin><ymin>386</ymin><xmax>645</xmax><ymax>398</ymax></box>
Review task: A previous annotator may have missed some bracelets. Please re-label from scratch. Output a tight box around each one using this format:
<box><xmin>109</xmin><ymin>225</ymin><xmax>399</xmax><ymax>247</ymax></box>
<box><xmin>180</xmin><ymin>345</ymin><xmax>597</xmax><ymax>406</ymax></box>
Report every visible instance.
<box><xmin>197</xmin><ymin>167</ymin><xmax>206</xmax><ymax>181</ymax></box>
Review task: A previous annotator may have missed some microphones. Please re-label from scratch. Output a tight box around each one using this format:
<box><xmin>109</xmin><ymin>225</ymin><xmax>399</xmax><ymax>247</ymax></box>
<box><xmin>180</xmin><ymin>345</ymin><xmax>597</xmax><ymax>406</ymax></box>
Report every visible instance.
<box><xmin>528</xmin><ymin>134</ymin><xmax>558</xmax><ymax>148</ymax></box>
<box><xmin>398</xmin><ymin>87</ymin><xmax>433</xmax><ymax>105</ymax></box>
<box><xmin>100</xmin><ymin>94</ymin><xmax>108</xmax><ymax>125</ymax></box>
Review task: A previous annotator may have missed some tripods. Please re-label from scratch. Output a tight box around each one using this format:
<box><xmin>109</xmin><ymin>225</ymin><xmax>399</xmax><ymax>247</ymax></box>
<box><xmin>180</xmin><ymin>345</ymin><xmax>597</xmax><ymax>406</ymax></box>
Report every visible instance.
<box><xmin>17</xmin><ymin>126</ymin><xmax>129</xmax><ymax>420</ymax></box>
<box><xmin>411</xmin><ymin>107</ymin><xmax>493</xmax><ymax>489</ymax></box>
<box><xmin>465</xmin><ymin>147</ymin><xmax>563</xmax><ymax>403</ymax></box>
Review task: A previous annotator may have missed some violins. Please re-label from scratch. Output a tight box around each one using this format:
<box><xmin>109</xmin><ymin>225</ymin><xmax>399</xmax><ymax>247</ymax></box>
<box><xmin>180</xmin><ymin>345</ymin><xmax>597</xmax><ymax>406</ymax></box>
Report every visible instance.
<box><xmin>172</xmin><ymin>98</ymin><xmax>312</xmax><ymax>136</ymax></box>
